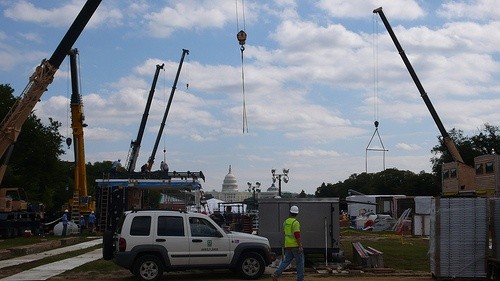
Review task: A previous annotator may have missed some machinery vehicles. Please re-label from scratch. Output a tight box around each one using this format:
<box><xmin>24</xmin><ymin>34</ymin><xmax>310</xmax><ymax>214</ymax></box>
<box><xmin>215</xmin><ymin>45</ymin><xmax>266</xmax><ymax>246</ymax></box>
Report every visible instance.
<box><xmin>0</xmin><ymin>1</ymin><xmax>249</xmax><ymax>240</ymax></box>
<box><xmin>144</xmin><ymin>47</ymin><xmax>190</xmax><ymax>171</ymax></box>
<box><xmin>116</xmin><ymin>63</ymin><xmax>165</xmax><ymax>176</ymax></box>
<box><xmin>369</xmin><ymin>5</ymin><xmax>500</xmax><ymax>197</ymax></box>
<box><xmin>65</xmin><ymin>47</ymin><xmax>97</xmax><ymax>225</ymax></box>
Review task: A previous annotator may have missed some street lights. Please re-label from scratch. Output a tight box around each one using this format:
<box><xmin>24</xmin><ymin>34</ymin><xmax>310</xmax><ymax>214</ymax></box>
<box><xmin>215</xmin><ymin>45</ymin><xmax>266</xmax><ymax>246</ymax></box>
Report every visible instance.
<box><xmin>270</xmin><ymin>167</ymin><xmax>289</xmax><ymax>196</ymax></box>
<box><xmin>247</xmin><ymin>181</ymin><xmax>261</xmax><ymax>200</ymax></box>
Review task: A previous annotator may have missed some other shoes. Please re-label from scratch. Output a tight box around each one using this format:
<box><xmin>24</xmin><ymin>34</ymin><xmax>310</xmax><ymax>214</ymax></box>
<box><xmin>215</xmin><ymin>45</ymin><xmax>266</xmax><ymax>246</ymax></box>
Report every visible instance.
<box><xmin>272</xmin><ymin>272</ymin><xmax>278</xmax><ymax>281</ymax></box>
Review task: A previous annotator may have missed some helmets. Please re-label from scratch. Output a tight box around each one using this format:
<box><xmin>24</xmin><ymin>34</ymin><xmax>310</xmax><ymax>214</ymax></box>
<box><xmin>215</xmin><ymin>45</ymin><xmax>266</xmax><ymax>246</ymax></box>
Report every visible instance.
<box><xmin>290</xmin><ymin>205</ymin><xmax>299</xmax><ymax>214</ymax></box>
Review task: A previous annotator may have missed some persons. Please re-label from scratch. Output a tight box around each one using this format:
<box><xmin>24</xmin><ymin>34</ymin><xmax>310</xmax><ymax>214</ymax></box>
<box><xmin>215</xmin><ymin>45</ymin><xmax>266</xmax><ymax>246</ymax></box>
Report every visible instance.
<box><xmin>79</xmin><ymin>215</ymin><xmax>85</xmax><ymax>232</ymax></box>
<box><xmin>88</xmin><ymin>210</ymin><xmax>96</xmax><ymax>233</ymax></box>
<box><xmin>62</xmin><ymin>209</ymin><xmax>68</xmax><ymax>237</ymax></box>
<box><xmin>112</xmin><ymin>159</ymin><xmax>121</xmax><ymax>178</ymax></box>
<box><xmin>371</xmin><ymin>210</ymin><xmax>375</xmax><ymax>215</ymax></box>
<box><xmin>141</xmin><ymin>163</ymin><xmax>147</xmax><ymax>172</ymax></box>
<box><xmin>272</xmin><ymin>205</ymin><xmax>305</xmax><ymax>281</ymax></box>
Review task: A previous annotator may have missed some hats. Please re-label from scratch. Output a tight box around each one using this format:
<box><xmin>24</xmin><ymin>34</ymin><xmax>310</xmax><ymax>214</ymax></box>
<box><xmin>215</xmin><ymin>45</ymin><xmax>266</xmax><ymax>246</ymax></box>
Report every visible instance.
<box><xmin>92</xmin><ymin>211</ymin><xmax>94</xmax><ymax>214</ymax></box>
<box><xmin>81</xmin><ymin>215</ymin><xmax>83</xmax><ymax>218</ymax></box>
<box><xmin>65</xmin><ymin>209</ymin><xmax>68</xmax><ymax>212</ymax></box>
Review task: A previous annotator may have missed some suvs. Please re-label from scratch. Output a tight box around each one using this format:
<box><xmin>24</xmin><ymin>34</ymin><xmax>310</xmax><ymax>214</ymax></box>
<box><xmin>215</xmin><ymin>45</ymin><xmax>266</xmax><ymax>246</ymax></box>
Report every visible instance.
<box><xmin>102</xmin><ymin>204</ymin><xmax>277</xmax><ymax>281</ymax></box>
